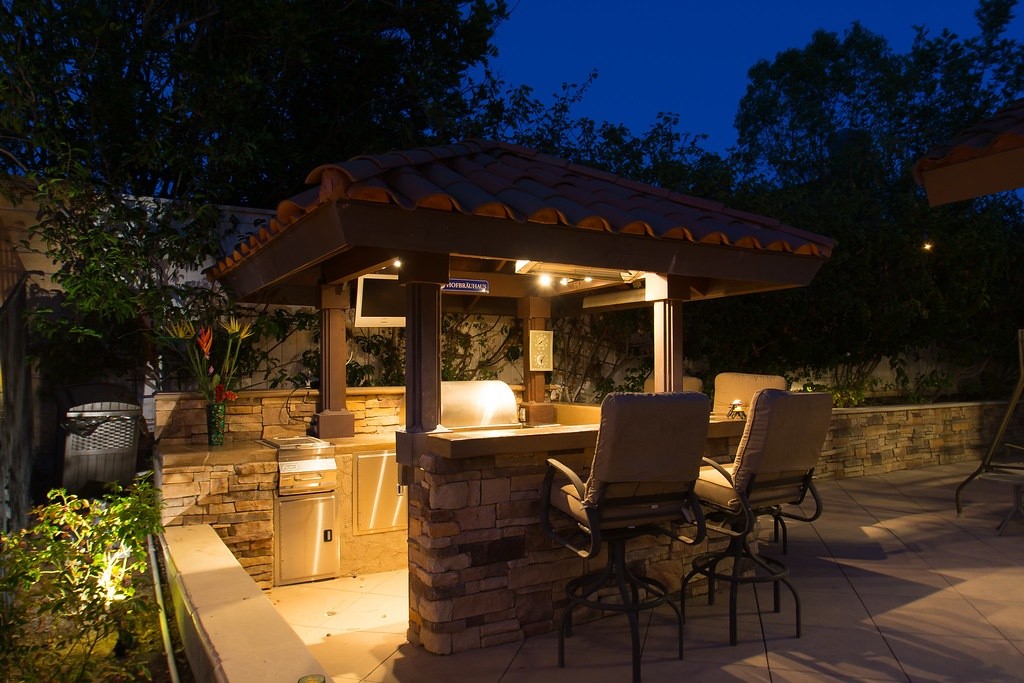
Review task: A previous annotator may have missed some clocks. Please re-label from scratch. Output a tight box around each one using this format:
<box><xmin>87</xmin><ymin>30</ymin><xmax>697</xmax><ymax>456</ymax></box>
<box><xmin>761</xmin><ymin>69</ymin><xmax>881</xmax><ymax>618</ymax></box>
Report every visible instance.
<box><xmin>529</xmin><ymin>330</ymin><xmax>553</xmax><ymax>371</ymax></box>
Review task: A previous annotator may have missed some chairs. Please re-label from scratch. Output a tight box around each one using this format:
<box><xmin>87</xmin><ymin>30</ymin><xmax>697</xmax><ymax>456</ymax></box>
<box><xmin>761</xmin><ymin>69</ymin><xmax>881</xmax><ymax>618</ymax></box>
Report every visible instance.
<box><xmin>540</xmin><ymin>392</ymin><xmax>711</xmax><ymax>683</ymax></box>
<box><xmin>645</xmin><ymin>372</ymin><xmax>833</xmax><ymax>644</ymax></box>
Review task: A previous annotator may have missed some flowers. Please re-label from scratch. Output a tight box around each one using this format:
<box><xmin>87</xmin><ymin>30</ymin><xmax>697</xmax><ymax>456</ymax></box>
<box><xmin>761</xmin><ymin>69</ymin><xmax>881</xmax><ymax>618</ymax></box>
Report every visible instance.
<box><xmin>148</xmin><ymin>316</ymin><xmax>259</xmax><ymax>403</ymax></box>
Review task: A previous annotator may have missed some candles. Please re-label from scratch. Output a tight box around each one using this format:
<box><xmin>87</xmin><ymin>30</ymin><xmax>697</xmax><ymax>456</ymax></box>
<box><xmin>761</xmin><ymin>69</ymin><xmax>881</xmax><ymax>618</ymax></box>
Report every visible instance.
<box><xmin>727</xmin><ymin>399</ymin><xmax>747</xmax><ymax>419</ymax></box>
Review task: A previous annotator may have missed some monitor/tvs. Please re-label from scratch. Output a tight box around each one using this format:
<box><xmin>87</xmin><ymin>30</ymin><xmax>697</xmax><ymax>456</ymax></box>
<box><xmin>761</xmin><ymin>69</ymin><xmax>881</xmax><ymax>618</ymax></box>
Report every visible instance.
<box><xmin>355</xmin><ymin>274</ymin><xmax>407</xmax><ymax>327</ymax></box>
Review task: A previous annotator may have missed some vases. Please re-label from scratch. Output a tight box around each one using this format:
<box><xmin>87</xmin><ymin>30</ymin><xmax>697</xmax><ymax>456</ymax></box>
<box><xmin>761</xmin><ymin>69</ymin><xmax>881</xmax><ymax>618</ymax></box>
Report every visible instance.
<box><xmin>206</xmin><ymin>404</ymin><xmax>225</xmax><ymax>446</ymax></box>
